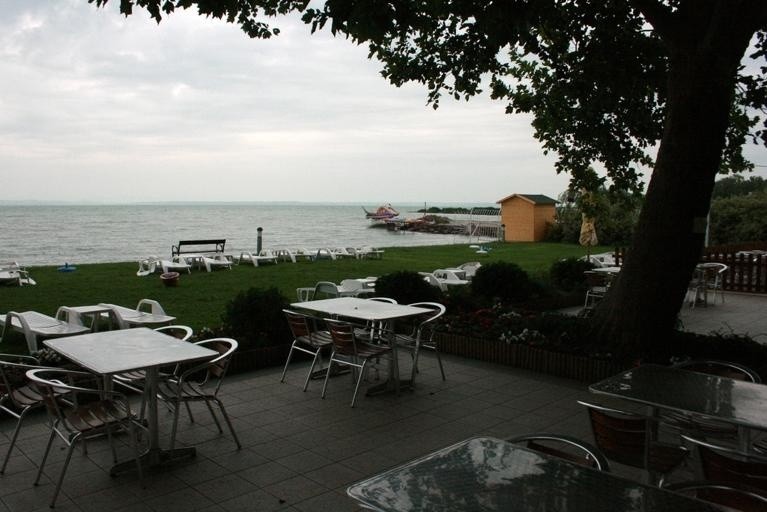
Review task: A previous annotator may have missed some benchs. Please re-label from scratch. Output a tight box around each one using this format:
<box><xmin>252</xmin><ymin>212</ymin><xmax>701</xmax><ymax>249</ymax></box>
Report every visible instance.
<box><xmin>171</xmin><ymin>239</ymin><xmax>227</xmax><ymax>260</ymax></box>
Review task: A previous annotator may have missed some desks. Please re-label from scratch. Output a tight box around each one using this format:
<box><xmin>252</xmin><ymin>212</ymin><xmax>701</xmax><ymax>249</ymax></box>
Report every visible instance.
<box><xmin>1</xmin><ymin>298</ymin><xmax>176</xmax><ymax>354</ymax></box>
<box><xmin>43</xmin><ymin>325</ymin><xmax>221</xmax><ymax>478</ymax></box>
<box><xmin>344</xmin><ymin>435</ymin><xmax>743</xmax><ymax>512</ymax></box>
<box><xmin>587</xmin><ymin>361</ymin><xmax>765</xmax><ymax>452</ymax></box>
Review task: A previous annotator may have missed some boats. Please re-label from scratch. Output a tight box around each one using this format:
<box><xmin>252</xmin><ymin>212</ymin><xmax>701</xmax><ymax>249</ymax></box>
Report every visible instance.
<box><xmin>360</xmin><ymin>203</ymin><xmax>399</xmax><ymax>221</ymax></box>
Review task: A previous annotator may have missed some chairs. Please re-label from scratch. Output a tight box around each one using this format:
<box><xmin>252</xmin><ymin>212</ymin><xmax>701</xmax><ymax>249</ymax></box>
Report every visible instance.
<box><xmin>153</xmin><ymin>335</ymin><xmax>240</xmax><ymax>463</ymax></box>
<box><xmin>572</xmin><ymin>397</ymin><xmax>690</xmax><ymax>488</ymax></box>
<box><xmin>1</xmin><ymin>258</ymin><xmax>41</xmax><ymax>292</ymax></box>
<box><xmin>284</xmin><ymin>262</ymin><xmax>482</xmax><ymax>409</ymax></box>
<box><xmin>575</xmin><ymin>258</ymin><xmax>732</xmax><ymax>320</ymax></box>
<box><xmin>115</xmin><ymin>325</ymin><xmax>195</xmax><ymax>428</ymax></box>
<box><xmin>676</xmin><ymin>484</ymin><xmax>766</xmax><ymax>512</ymax></box>
<box><xmin>505</xmin><ymin>433</ymin><xmax>611</xmax><ymax>474</ymax></box>
<box><xmin>2</xmin><ymin>350</ymin><xmax>155</xmax><ymax>466</ymax></box>
<box><xmin>129</xmin><ymin>244</ymin><xmax>386</xmax><ymax>279</ymax></box>
<box><xmin>25</xmin><ymin>367</ymin><xmax>149</xmax><ymax>510</ymax></box>
<box><xmin>677</xmin><ymin>357</ymin><xmax>762</xmax><ymax>385</ymax></box>
<box><xmin>681</xmin><ymin>436</ymin><xmax>766</xmax><ymax>497</ymax></box>
